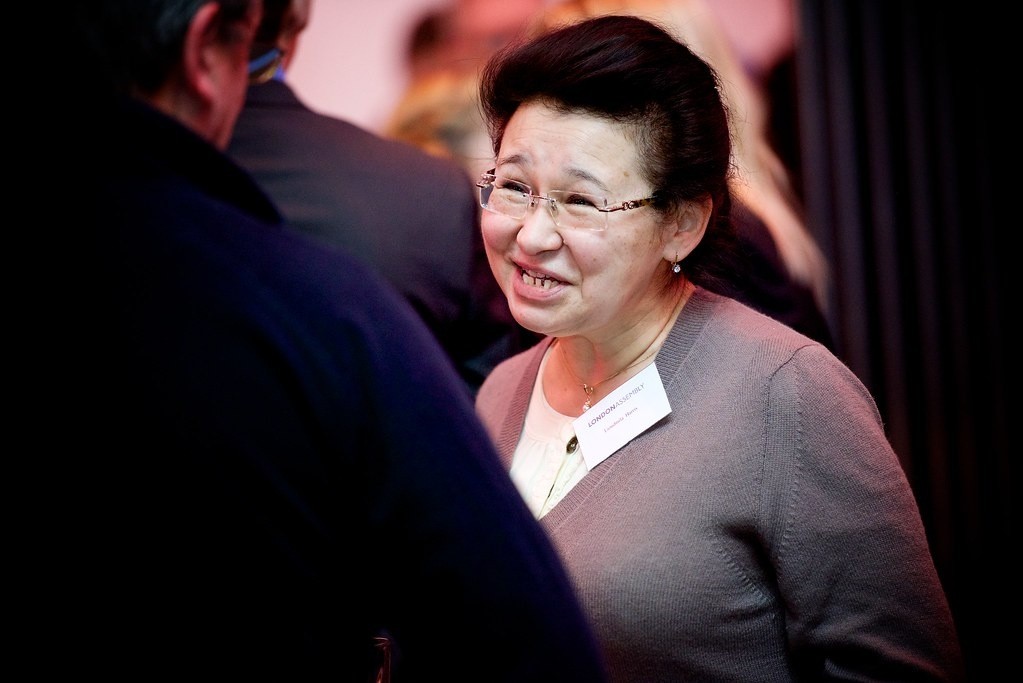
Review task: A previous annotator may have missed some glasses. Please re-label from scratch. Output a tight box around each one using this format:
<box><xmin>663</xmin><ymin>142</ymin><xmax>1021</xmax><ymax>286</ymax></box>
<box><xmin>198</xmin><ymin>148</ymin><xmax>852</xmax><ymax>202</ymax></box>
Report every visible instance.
<box><xmin>475</xmin><ymin>168</ymin><xmax>677</xmax><ymax>232</ymax></box>
<box><xmin>219</xmin><ymin>9</ymin><xmax>285</xmax><ymax>84</ymax></box>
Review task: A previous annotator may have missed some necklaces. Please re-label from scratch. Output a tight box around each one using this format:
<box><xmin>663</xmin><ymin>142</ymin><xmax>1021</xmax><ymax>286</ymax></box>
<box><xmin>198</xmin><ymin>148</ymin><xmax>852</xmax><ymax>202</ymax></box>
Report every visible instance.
<box><xmin>582</xmin><ymin>342</ymin><xmax>661</xmax><ymax>412</ymax></box>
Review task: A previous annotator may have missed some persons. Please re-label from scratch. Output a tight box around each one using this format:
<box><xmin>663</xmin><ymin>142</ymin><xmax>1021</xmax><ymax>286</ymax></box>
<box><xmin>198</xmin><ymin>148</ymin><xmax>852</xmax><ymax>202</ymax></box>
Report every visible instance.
<box><xmin>0</xmin><ymin>0</ymin><xmax>604</xmax><ymax>683</ymax></box>
<box><xmin>471</xmin><ymin>15</ymin><xmax>958</xmax><ymax>683</ymax></box>
<box><xmin>226</xmin><ymin>0</ymin><xmax>492</xmax><ymax>369</ymax></box>
